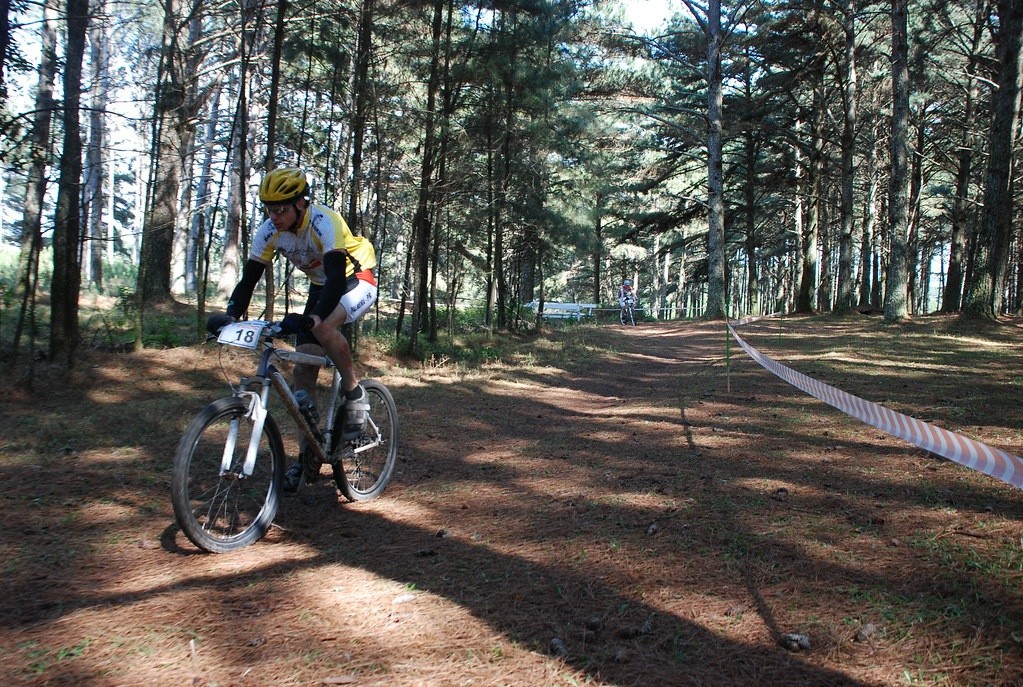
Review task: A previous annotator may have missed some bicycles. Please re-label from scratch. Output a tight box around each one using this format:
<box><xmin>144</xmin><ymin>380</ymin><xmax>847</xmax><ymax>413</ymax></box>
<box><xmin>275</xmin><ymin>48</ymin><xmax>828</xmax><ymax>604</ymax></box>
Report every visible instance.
<box><xmin>619</xmin><ymin>297</ymin><xmax>639</xmax><ymax>327</ymax></box>
<box><xmin>170</xmin><ymin>318</ymin><xmax>401</xmax><ymax>554</ymax></box>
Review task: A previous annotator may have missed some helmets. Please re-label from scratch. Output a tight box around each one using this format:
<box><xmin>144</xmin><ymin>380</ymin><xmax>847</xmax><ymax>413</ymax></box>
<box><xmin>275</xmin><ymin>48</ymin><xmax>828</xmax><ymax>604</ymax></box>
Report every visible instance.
<box><xmin>624</xmin><ymin>280</ymin><xmax>630</xmax><ymax>286</ymax></box>
<box><xmin>259</xmin><ymin>166</ymin><xmax>306</xmax><ymax>204</ymax></box>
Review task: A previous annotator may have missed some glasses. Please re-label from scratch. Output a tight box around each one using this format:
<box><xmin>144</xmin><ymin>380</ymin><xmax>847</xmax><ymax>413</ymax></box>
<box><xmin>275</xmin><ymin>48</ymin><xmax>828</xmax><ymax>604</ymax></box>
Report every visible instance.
<box><xmin>264</xmin><ymin>198</ymin><xmax>307</xmax><ymax>217</ymax></box>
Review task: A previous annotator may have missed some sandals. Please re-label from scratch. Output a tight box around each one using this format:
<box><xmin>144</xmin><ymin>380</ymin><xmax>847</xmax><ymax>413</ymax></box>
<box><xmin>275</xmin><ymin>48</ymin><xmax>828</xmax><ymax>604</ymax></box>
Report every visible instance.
<box><xmin>281</xmin><ymin>463</ymin><xmax>319</xmax><ymax>487</ymax></box>
<box><xmin>342</xmin><ymin>384</ymin><xmax>371</xmax><ymax>440</ymax></box>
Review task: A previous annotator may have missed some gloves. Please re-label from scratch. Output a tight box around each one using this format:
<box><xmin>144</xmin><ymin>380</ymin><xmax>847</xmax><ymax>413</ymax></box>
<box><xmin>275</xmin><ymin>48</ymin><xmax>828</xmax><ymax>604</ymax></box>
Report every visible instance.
<box><xmin>282</xmin><ymin>313</ymin><xmax>315</xmax><ymax>333</ymax></box>
<box><xmin>206</xmin><ymin>315</ymin><xmax>229</xmax><ymax>337</ymax></box>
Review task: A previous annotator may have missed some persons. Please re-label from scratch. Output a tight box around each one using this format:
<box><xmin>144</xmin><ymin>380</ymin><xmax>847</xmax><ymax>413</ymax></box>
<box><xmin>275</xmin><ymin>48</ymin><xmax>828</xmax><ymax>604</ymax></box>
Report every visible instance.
<box><xmin>204</xmin><ymin>167</ymin><xmax>378</xmax><ymax>489</ymax></box>
<box><xmin>617</xmin><ymin>280</ymin><xmax>633</xmax><ymax>319</ymax></box>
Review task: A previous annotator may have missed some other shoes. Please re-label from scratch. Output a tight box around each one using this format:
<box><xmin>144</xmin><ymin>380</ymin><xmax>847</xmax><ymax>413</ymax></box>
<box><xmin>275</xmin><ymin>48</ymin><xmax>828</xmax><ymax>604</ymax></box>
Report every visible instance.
<box><xmin>622</xmin><ymin>319</ymin><xmax>626</xmax><ymax>322</ymax></box>
